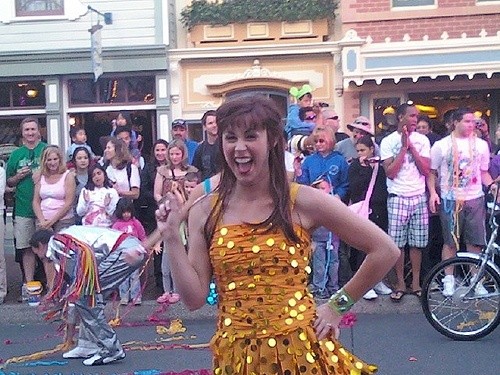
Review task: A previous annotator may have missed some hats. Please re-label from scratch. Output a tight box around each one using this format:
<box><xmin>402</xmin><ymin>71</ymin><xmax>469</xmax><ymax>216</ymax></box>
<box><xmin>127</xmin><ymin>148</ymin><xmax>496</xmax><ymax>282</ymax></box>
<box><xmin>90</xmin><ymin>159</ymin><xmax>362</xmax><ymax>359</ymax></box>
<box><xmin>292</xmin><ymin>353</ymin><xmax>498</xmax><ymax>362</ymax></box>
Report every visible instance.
<box><xmin>347</xmin><ymin>117</ymin><xmax>375</xmax><ymax>137</ymax></box>
<box><xmin>172</xmin><ymin>119</ymin><xmax>188</xmax><ymax>128</ymax></box>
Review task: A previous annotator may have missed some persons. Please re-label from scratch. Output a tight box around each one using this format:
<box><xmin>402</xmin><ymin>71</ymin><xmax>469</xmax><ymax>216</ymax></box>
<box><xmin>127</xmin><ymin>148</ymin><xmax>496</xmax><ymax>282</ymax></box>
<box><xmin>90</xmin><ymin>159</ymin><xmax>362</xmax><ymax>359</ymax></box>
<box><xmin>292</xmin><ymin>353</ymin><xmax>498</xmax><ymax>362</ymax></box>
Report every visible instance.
<box><xmin>155</xmin><ymin>94</ymin><xmax>401</xmax><ymax>375</ymax></box>
<box><xmin>283</xmin><ymin>85</ymin><xmax>500</xmax><ymax>300</ymax></box>
<box><xmin>29</xmin><ymin>225</ymin><xmax>149</xmax><ymax>365</ymax></box>
<box><xmin>0</xmin><ymin>109</ymin><xmax>222</xmax><ymax>309</ymax></box>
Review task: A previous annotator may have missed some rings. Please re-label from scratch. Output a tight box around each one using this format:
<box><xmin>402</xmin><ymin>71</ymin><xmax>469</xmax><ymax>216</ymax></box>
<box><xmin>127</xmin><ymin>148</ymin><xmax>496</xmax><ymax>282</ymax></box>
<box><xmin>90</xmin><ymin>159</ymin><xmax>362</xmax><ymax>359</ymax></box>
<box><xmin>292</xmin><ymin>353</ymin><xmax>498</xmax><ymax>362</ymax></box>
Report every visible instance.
<box><xmin>327</xmin><ymin>323</ymin><xmax>332</xmax><ymax>328</ymax></box>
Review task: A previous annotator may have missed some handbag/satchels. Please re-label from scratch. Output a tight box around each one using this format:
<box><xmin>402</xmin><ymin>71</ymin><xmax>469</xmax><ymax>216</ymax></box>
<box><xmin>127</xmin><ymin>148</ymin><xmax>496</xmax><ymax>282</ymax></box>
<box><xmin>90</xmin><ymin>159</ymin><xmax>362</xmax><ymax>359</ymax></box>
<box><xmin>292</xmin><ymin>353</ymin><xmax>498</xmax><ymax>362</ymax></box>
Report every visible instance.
<box><xmin>348</xmin><ymin>200</ymin><xmax>372</xmax><ymax>220</ymax></box>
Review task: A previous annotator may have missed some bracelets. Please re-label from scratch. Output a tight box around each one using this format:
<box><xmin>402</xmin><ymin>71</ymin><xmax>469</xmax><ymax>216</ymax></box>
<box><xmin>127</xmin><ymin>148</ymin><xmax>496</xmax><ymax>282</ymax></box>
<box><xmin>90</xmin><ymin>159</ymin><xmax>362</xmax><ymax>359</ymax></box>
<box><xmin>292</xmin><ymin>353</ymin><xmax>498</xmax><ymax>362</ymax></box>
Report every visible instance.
<box><xmin>329</xmin><ymin>287</ymin><xmax>356</xmax><ymax>314</ymax></box>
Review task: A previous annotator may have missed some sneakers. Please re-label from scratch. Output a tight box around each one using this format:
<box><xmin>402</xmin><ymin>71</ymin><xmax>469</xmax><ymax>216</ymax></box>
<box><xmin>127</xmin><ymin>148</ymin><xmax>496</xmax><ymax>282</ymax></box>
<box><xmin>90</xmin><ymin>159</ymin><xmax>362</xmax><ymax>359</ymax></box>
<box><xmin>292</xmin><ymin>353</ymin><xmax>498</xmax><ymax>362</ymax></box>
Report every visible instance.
<box><xmin>442</xmin><ymin>275</ymin><xmax>455</xmax><ymax>296</ymax></box>
<box><xmin>470</xmin><ymin>277</ymin><xmax>488</xmax><ymax>294</ymax></box>
<box><xmin>63</xmin><ymin>345</ymin><xmax>100</xmax><ymax>359</ymax></box>
<box><xmin>82</xmin><ymin>348</ymin><xmax>126</xmax><ymax>366</ymax></box>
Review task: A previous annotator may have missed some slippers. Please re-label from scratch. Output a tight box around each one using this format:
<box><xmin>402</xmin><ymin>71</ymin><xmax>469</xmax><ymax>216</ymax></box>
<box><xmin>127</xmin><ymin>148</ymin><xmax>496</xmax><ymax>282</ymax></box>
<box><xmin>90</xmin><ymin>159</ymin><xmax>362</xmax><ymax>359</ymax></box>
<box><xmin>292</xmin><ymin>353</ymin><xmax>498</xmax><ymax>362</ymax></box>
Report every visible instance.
<box><xmin>410</xmin><ymin>290</ymin><xmax>431</xmax><ymax>299</ymax></box>
<box><xmin>390</xmin><ymin>291</ymin><xmax>408</xmax><ymax>302</ymax></box>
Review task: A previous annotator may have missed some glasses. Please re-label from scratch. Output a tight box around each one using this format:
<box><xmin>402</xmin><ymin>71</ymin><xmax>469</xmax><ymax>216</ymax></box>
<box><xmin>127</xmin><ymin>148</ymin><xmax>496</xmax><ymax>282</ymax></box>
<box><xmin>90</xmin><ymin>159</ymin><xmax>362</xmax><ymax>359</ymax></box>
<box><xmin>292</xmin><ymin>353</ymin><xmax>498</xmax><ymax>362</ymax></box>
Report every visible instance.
<box><xmin>328</xmin><ymin>116</ymin><xmax>338</xmax><ymax>120</ymax></box>
<box><xmin>29</xmin><ymin>149</ymin><xmax>35</xmax><ymax>164</ymax></box>
<box><xmin>314</xmin><ymin>139</ymin><xmax>324</xmax><ymax>143</ymax></box>
<box><xmin>302</xmin><ymin>115</ymin><xmax>316</xmax><ymax>120</ymax></box>
<box><xmin>172</xmin><ymin>120</ymin><xmax>186</xmax><ymax>126</ymax></box>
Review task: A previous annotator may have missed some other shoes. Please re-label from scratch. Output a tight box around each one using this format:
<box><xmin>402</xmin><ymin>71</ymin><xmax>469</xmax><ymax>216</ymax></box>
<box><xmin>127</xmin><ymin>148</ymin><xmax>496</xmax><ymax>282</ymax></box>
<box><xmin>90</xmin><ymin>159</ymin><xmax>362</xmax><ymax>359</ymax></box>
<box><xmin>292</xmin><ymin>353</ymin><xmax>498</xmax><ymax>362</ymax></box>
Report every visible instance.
<box><xmin>120</xmin><ymin>297</ymin><xmax>129</xmax><ymax>305</ymax></box>
<box><xmin>373</xmin><ymin>281</ymin><xmax>392</xmax><ymax>294</ymax></box>
<box><xmin>315</xmin><ymin>287</ymin><xmax>340</xmax><ymax>299</ymax></box>
<box><xmin>169</xmin><ymin>293</ymin><xmax>180</xmax><ymax>304</ymax></box>
<box><xmin>363</xmin><ymin>289</ymin><xmax>378</xmax><ymax>300</ymax></box>
<box><xmin>157</xmin><ymin>292</ymin><xmax>172</xmax><ymax>303</ymax></box>
<box><xmin>133</xmin><ymin>298</ymin><xmax>142</xmax><ymax>305</ymax></box>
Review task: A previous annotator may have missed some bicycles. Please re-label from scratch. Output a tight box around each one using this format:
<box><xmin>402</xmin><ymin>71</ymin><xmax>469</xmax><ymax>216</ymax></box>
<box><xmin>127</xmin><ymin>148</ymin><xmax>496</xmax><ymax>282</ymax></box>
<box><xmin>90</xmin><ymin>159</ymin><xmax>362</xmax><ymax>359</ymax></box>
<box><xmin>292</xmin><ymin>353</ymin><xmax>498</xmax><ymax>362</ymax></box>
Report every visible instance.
<box><xmin>420</xmin><ymin>178</ymin><xmax>500</xmax><ymax>341</ymax></box>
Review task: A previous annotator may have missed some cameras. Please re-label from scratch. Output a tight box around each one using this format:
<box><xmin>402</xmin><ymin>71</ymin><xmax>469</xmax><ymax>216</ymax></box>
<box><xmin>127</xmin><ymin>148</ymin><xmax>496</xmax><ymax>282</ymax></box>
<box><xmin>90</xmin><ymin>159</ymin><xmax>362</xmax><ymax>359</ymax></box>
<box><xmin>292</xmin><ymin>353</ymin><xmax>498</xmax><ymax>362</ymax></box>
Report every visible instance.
<box><xmin>297</xmin><ymin>156</ymin><xmax>303</xmax><ymax>162</ymax></box>
